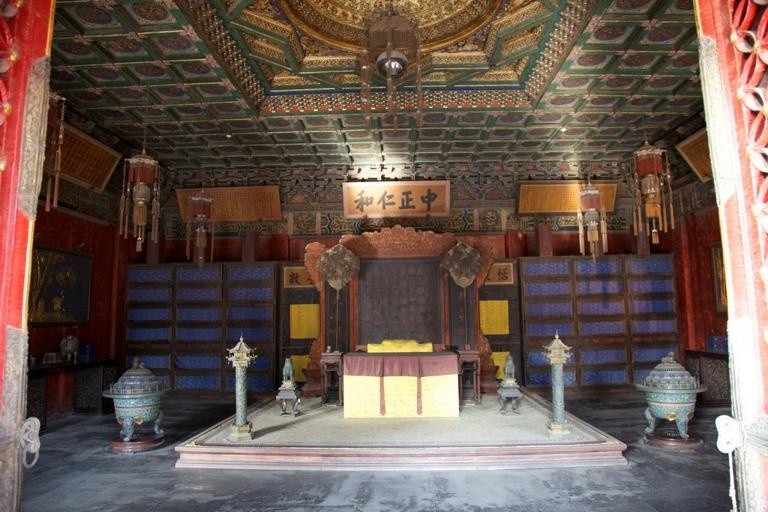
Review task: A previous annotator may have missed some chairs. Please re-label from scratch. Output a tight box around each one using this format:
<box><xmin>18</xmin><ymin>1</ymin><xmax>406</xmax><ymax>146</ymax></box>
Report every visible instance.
<box><xmin>365</xmin><ymin>338</ymin><xmax>435</xmax><ymax>353</ymax></box>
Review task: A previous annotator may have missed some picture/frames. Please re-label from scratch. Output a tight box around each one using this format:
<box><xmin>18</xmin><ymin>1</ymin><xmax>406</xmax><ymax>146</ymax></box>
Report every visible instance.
<box><xmin>709</xmin><ymin>237</ymin><xmax>730</xmax><ymax>318</ymax></box>
<box><xmin>28</xmin><ymin>240</ymin><xmax>94</xmax><ymax>330</ymax></box>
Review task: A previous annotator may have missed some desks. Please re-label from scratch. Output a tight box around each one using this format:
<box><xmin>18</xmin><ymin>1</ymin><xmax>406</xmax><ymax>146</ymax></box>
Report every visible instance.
<box><xmin>28</xmin><ymin>356</ymin><xmax>122</xmax><ymax>436</ymax></box>
<box><xmin>683</xmin><ymin>347</ymin><xmax>730</xmax><ymax>410</ymax></box>
<box><xmin>339</xmin><ymin>350</ymin><xmax>466</xmax><ymax>421</ymax></box>
<box><xmin>455</xmin><ymin>348</ymin><xmax>484</xmax><ymax>407</ymax></box>
<box><xmin>320</xmin><ymin>350</ymin><xmax>345</xmax><ymax>406</ymax></box>
